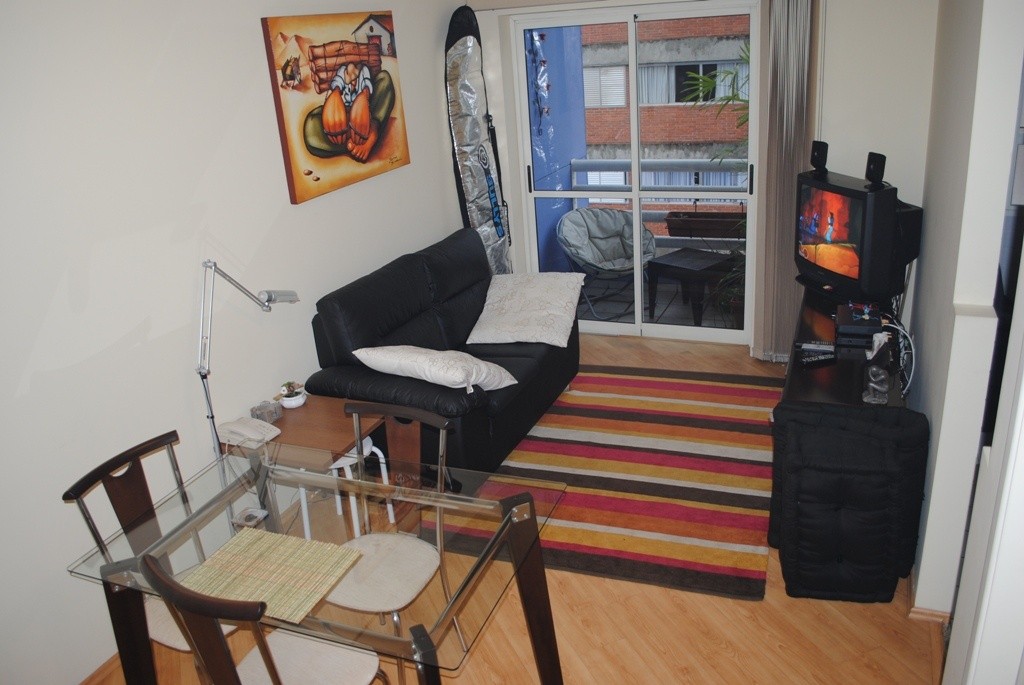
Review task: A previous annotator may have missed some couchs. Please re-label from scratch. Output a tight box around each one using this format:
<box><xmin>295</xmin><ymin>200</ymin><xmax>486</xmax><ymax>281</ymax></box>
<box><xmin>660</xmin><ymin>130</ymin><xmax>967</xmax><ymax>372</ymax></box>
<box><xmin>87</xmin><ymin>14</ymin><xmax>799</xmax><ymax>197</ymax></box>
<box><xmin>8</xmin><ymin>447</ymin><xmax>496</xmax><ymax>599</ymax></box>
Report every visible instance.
<box><xmin>304</xmin><ymin>227</ymin><xmax>581</xmax><ymax>496</ymax></box>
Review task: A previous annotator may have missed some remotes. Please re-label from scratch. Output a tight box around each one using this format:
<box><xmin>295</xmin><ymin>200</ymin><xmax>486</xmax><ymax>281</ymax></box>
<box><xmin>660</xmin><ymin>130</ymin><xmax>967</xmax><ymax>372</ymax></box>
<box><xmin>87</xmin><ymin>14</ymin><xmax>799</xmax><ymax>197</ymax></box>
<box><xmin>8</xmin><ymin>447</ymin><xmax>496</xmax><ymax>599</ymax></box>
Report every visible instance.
<box><xmin>794</xmin><ymin>339</ymin><xmax>836</xmax><ymax>364</ymax></box>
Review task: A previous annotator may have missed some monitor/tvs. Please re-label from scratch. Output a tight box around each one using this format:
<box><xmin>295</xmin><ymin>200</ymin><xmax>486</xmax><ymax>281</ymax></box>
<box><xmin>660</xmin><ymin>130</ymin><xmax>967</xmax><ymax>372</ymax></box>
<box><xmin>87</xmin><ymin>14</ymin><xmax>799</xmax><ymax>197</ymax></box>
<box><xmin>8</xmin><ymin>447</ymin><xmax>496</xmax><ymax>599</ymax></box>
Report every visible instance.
<box><xmin>795</xmin><ymin>170</ymin><xmax>924</xmax><ymax>305</ymax></box>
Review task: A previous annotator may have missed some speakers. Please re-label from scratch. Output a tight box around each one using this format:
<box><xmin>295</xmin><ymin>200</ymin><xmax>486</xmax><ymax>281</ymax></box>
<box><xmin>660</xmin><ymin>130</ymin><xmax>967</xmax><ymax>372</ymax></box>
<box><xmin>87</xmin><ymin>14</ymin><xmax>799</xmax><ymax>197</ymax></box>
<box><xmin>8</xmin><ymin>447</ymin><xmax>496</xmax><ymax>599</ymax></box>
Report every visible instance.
<box><xmin>810</xmin><ymin>141</ymin><xmax>828</xmax><ymax>174</ymax></box>
<box><xmin>864</xmin><ymin>152</ymin><xmax>886</xmax><ymax>190</ymax></box>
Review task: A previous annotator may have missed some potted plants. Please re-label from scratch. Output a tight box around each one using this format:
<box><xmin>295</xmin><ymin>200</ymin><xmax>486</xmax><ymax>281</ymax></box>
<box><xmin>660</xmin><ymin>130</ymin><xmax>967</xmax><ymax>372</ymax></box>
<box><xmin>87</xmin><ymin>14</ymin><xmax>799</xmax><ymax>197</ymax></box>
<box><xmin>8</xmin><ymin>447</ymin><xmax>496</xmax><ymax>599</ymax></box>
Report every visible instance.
<box><xmin>701</xmin><ymin>217</ymin><xmax>746</xmax><ymax>330</ymax></box>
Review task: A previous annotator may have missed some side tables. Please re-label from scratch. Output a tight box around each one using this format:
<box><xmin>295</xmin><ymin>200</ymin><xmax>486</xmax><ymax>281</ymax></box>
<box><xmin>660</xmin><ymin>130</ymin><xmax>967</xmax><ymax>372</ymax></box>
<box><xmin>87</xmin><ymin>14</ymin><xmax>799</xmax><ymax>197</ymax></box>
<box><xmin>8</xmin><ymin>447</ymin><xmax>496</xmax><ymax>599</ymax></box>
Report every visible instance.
<box><xmin>214</xmin><ymin>394</ymin><xmax>385</xmax><ymax>545</ymax></box>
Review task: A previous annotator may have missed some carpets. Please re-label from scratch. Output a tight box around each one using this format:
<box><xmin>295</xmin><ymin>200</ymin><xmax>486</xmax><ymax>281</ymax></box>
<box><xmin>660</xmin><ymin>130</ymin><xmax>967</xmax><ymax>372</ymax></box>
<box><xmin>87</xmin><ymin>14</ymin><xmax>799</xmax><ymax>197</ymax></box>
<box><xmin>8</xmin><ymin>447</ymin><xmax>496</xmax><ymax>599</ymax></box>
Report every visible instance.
<box><xmin>422</xmin><ymin>364</ymin><xmax>787</xmax><ymax>600</ymax></box>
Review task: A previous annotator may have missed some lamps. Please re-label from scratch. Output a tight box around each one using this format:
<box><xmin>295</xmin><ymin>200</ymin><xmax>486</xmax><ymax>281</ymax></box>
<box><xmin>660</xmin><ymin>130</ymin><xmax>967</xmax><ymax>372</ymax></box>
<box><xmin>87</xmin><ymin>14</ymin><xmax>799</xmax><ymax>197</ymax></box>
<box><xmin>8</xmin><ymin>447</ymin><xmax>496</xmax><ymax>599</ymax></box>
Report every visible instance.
<box><xmin>196</xmin><ymin>259</ymin><xmax>303</xmax><ymax>535</ymax></box>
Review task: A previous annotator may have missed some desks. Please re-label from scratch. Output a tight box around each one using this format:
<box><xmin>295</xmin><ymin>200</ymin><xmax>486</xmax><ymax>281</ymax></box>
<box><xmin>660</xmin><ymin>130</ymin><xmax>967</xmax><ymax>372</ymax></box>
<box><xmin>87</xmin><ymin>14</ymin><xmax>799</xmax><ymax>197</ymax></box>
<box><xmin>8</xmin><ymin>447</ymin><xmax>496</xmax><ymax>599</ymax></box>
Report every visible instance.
<box><xmin>66</xmin><ymin>434</ymin><xmax>570</xmax><ymax>685</ymax></box>
<box><xmin>647</xmin><ymin>247</ymin><xmax>735</xmax><ymax>325</ymax></box>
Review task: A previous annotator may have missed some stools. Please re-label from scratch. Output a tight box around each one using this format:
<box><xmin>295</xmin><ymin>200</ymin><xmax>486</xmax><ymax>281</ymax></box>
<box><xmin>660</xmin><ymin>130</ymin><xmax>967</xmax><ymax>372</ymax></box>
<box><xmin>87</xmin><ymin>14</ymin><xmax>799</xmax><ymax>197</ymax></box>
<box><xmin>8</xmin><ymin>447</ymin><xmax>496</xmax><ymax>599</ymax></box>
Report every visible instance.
<box><xmin>299</xmin><ymin>436</ymin><xmax>395</xmax><ymax>539</ymax></box>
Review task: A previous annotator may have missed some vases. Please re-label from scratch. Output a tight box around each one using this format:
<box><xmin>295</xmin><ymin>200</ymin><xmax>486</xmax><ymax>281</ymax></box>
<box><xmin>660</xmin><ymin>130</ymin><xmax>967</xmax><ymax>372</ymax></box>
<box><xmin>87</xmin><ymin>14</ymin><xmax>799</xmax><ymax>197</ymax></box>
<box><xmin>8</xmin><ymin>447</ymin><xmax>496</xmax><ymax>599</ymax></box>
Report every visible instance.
<box><xmin>664</xmin><ymin>211</ymin><xmax>746</xmax><ymax>238</ymax></box>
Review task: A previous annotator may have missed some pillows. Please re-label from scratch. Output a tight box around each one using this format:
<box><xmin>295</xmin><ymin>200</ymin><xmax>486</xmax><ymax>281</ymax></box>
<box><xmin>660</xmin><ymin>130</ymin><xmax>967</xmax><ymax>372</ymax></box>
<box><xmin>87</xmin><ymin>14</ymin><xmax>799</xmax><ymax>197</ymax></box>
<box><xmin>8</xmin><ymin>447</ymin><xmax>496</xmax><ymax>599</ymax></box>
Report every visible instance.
<box><xmin>352</xmin><ymin>345</ymin><xmax>518</xmax><ymax>395</ymax></box>
<box><xmin>466</xmin><ymin>271</ymin><xmax>587</xmax><ymax>348</ymax></box>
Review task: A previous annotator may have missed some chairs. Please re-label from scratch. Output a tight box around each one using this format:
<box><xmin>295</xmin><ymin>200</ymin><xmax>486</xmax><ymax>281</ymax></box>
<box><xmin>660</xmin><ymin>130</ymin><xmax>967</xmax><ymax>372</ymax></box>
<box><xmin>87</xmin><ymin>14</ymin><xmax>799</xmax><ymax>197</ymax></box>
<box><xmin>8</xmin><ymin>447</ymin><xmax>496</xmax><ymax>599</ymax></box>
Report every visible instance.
<box><xmin>61</xmin><ymin>429</ymin><xmax>239</xmax><ymax>685</ymax></box>
<box><xmin>555</xmin><ymin>206</ymin><xmax>656</xmax><ymax>320</ymax></box>
<box><xmin>140</xmin><ymin>554</ymin><xmax>392</xmax><ymax>685</ymax></box>
<box><xmin>323</xmin><ymin>401</ymin><xmax>469</xmax><ymax>685</ymax></box>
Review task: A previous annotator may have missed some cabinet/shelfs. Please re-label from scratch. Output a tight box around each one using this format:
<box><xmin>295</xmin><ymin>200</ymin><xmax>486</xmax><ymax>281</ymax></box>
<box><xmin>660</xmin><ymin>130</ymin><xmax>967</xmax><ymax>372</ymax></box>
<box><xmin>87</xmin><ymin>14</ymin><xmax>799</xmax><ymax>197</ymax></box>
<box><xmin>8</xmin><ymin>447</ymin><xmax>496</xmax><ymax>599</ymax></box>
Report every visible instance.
<box><xmin>782</xmin><ymin>289</ymin><xmax>905</xmax><ymax>407</ymax></box>
